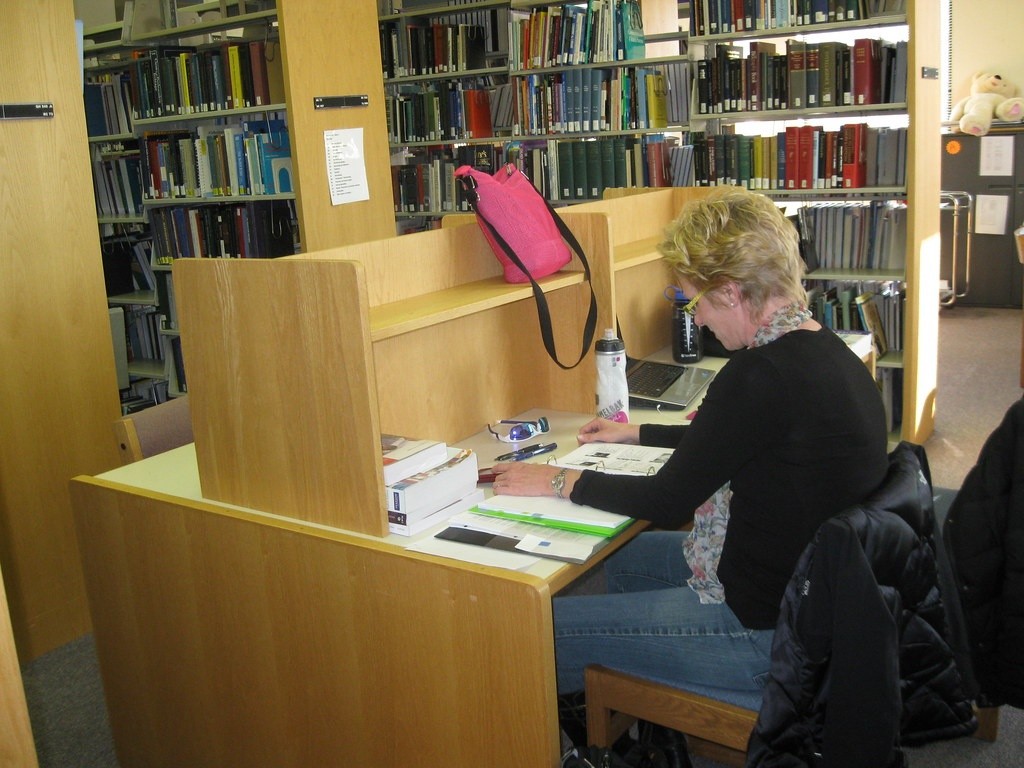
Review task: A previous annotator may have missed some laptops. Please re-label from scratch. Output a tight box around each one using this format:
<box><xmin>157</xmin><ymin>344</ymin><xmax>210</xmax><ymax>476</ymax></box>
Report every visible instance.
<box><xmin>616</xmin><ymin>317</ymin><xmax>716</xmax><ymax>411</ymax></box>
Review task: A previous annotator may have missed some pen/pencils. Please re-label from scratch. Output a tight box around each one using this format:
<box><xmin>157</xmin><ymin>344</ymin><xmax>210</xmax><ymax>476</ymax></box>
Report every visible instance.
<box><xmin>478</xmin><ymin>474</ymin><xmax>501</xmax><ymax>482</ymax></box>
<box><xmin>493</xmin><ymin>441</ymin><xmax>558</xmax><ymax>462</ymax></box>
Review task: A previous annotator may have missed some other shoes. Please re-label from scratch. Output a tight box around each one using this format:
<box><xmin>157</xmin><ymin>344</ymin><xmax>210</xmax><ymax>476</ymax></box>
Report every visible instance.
<box><xmin>643</xmin><ymin>723</ymin><xmax>693</xmax><ymax>768</ymax></box>
<box><xmin>617</xmin><ymin>739</ymin><xmax>666</xmax><ymax>768</ymax></box>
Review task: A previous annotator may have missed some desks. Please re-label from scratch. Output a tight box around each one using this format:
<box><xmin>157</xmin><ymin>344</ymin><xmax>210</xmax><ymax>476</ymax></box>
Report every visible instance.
<box><xmin>70</xmin><ymin>187</ymin><xmax>875</xmax><ymax>768</ymax></box>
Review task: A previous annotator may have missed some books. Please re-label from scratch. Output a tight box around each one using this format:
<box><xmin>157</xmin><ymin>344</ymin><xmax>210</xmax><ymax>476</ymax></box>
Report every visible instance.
<box><xmin>379</xmin><ymin>435</ymin><xmax>675</xmax><ymax>565</ymax></box>
<box><xmin>80</xmin><ymin>0</ymin><xmax>908</xmax><ymax>417</ymax></box>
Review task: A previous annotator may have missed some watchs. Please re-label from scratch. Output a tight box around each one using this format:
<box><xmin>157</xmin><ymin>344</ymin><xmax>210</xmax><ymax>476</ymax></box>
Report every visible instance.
<box><xmin>551</xmin><ymin>467</ymin><xmax>570</xmax><ymax>500</ymax></box>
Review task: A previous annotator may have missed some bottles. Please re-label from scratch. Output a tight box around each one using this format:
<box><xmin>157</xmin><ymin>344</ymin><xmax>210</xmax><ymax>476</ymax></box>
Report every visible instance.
<box><xmin>663</xmin><ymin>285</ymin><xmax>704</xmax><ymax>364</ymax></box>
<box><xmin>593</xmin><ymin>328</ymin><xmax>629</xmax><ymax>423</ymax></box>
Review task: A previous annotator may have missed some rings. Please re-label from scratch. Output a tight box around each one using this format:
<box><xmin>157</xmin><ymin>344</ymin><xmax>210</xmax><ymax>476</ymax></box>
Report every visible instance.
<box><xmin>497</xmin><ymin>481</ymin><xmax>501</xmax><ymax>487</ymax></box>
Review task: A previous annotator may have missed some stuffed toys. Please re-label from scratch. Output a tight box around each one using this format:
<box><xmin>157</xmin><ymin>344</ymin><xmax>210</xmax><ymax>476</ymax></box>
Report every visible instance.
<box><xmin>946</xmin><ymin>71</ymin><xmax>1024</xmax><ymax>137</ymax></box>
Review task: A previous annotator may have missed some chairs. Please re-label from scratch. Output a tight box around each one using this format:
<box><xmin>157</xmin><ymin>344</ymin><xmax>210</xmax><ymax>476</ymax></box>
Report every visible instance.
<box><xmin>585</xmin><ymin>398</ymin><xmax>1024</xmax><ymax>768</ymax></box>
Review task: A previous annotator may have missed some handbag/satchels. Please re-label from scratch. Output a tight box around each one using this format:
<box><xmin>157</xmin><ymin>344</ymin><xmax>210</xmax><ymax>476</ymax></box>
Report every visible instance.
<box><xmin>454</xmin><ymin>163</ymin><xmax>572</xmax><ymax>283</ymax></box>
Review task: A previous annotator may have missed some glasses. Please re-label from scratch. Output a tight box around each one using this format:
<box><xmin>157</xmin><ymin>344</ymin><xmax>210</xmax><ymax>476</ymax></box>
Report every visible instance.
<box><xmin>487</xmin><ymin>416</ymin><xmax>550</xmax><ymax>443</ymax></box>
<box><xmin>683</xmin><ymin>275</ymin><xmax>723</xmax><ymax>315</ymax></box>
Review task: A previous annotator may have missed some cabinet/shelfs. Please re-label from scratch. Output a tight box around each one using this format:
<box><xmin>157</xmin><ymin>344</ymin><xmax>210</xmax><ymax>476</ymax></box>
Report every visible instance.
<box><xmin>0</xmin><ymin>0</ymin><xmax>944</xmax><ymax>662</ymax></box>
<box><xmin>941</xmin><ymin>126</ymin><xmax>1024</xmax><ymax>307</ymax></box>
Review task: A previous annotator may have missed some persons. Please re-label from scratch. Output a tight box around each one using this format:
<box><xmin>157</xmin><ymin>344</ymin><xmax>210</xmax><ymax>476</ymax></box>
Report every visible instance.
<box><xmin>473</xmin><ymin>186</ymin><xmax>890</xmax><ymax>768</ymax></box>
